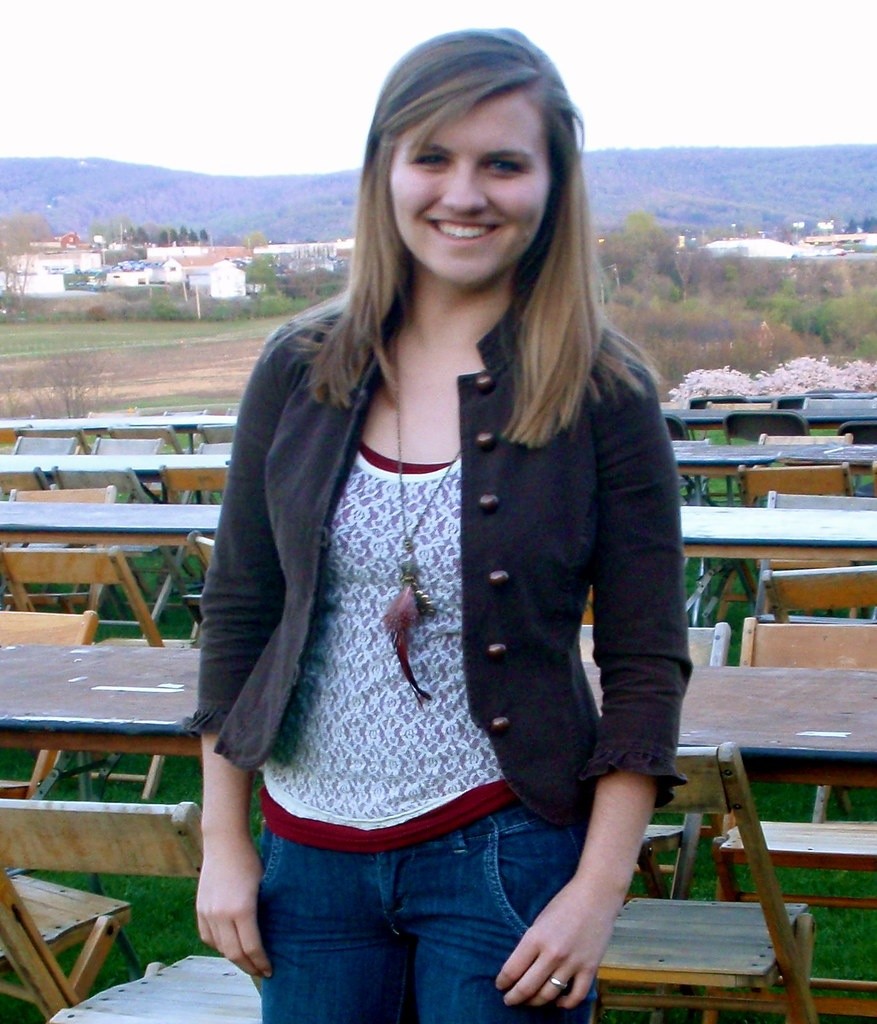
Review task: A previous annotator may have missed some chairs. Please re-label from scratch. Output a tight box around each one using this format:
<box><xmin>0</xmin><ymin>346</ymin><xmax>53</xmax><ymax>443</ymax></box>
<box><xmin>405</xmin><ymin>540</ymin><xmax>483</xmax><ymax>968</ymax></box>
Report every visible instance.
<box><xmin>0</xmin><ymin>391</ymin><xmax>876</xmax><ymax>1024</ymax></box>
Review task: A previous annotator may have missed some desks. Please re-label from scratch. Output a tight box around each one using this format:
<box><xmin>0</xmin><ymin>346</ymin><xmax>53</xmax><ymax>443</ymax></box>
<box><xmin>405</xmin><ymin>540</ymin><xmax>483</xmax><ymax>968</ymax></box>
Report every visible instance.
<box><xmin>670</xmin><ymin>444</ymin><xmax>846</xmax><ymax>618</ymax></box>
<box><xmin>0</xmin><ymin>417</ymin><xmax>237</xmax><ymax>455</ymax></box>
<box><xmin>678</xmin><ymin>505</ymin><xmax>877</xmax><ymax>628</ymax></box>
<box><xmin>0</xmin><ymin>642</ymin><xmax>203</xmax><ymax>985</ymax></box>
<box><xmin>0</xmin><ymin>453</ymin><xmax>230</xmax><ymax>504</ymax></box>
<box><xmin>662</xmin><ymin>407</ymin><xmax>877</xmax><ymax>432</ymax></box>
<box><xmin>577</xmin><ymin>656</ymin><xmax>877</xmax><ymax>786</ymax></box>
<box><xmin>0</xmin><ymin>500</ymin><xmax>222</xmax><ymax>639</ymax></box>
<box><xmin>776</xmin><ymin>441</ymin><xmax>877</xmax><ymax>493</ymax></box>
<box><xmin>747</xmin><ymin>393</ymin><xmax>877</xmax><ymax>403</ymax></box>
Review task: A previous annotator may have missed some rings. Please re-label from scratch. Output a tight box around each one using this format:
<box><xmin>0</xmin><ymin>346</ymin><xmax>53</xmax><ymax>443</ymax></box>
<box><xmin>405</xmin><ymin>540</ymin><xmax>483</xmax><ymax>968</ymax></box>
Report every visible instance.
<box><xmin>549</xmin><ymin>977</ymin><xmax>567</xmax><ymax>989</ymax></box>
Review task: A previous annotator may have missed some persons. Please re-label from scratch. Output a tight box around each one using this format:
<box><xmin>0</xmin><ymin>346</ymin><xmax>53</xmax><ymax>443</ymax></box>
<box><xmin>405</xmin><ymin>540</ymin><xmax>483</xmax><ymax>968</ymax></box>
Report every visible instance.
<box><xmin>191</xmin><ymin>31</ymin><xmax>692</xmax><ymax>1024</ymax></box>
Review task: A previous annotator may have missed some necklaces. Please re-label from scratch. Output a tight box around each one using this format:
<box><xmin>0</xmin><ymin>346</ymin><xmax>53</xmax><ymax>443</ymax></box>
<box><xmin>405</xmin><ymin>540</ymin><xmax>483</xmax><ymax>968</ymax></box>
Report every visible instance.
<box><xmin>388</xmin><ymin>342</ymin><xmax>460</xmax><ymax>701</ymax></box>
